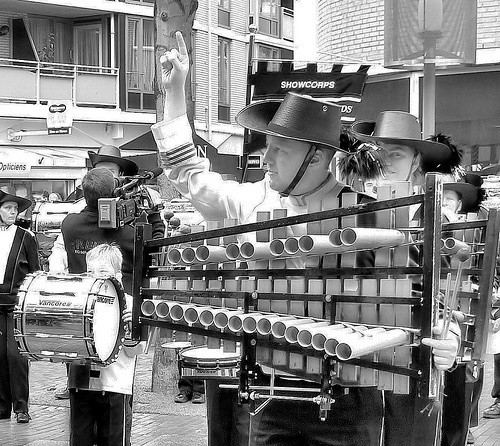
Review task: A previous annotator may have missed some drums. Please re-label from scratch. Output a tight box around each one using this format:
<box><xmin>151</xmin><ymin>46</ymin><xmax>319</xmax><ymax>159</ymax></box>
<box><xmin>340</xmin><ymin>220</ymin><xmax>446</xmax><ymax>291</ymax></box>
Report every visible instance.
<box><xmin>32</xmin><ymin>201</ymin><xmax>75</xmax><ymax>251</ymax></box>
<box><xmin>13</xmin><ymin>270</ymin><xmax>127</xmax><ymax>367</ymax></box>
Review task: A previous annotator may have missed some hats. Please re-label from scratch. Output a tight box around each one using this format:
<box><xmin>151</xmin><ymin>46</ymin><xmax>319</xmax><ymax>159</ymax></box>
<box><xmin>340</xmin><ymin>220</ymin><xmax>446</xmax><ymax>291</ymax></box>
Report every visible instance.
<box><xmin>419</xmin><ymin>172</ymin><xmax>477</xmax><ymax>213</ymax></box>
<box><xmin>234</xmin><ymin>92</ymin><xmax>358</xmax><ymax>197</ymax></box>
<box><xmin>350</xmin><ymin>111</ymin><xmax>452</xmax><ymax>182</ymax></box>
<box><xmin>87</xmin><ymin>145</ymin><xmax>138</xmax><ymax>180</ymax></box>
<box><xmin>0</xmin><ymin>186</ymin><xmax>32</xmax><ymax>215</ymax></box>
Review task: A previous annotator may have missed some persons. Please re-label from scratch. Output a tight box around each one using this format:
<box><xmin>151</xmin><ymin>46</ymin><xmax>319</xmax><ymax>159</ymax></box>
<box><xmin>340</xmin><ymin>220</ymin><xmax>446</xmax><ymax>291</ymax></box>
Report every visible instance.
<box><xmin>349</xmin><ymin>110</ymin><xmax>500</xmax><ymax>446</ymax></box>
<box><xmin>60</xmin><ymin>169</ymin><xmax>166</xmax><ymax>411</ymax></box>
<box><xmin>173</xmin><ymin>378</ymin><xmax>207</xmax><ymax>404</ymax></box>
<box><xmin>65</xmin><ymin>242</ymin><xmax>145</xmax><ymax>446</ymax></box>
<box><xmin>430</xmin><ymin>161</ymin><xmax>500</xmax><ymax>446</ymax></box>
<box><xmin>1</xmin><ymin>184</ymin><xmax>44</xmax><ymax>424</ymax></box>
<box><xmin>48</xmin><ymin>145</ymin><xmax>138</xmax><ymax>400</ymax></box>
<box><xmin>149</xmin><ymin>29</ymin><xmax>462</xmax><ymax>445</ymax></box>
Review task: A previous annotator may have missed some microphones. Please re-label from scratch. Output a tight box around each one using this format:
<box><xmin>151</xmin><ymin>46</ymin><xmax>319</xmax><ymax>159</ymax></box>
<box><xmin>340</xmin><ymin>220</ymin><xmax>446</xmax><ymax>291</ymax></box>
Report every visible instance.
<box><xmin>144</xmin><ymin>168</ymin><xmax>163</xmax><ymax>180</ymax></box>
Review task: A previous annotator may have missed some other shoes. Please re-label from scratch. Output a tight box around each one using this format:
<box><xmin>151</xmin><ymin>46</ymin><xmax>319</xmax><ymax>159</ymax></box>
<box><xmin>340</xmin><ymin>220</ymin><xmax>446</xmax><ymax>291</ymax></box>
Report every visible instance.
<box><xmin>174</xmin><ymin>392</ymin><xmax>190</xmax><ymax>402</ymax></box>
<box><xmin>484</xmin><ymin>398</ymin><xmax>500</xmax><ymax>418</ymax></box>
<box><xmin>15</xmin><ymin>413</ymin><xmax>31</xmax><ymax>423</ymax></box>
<box><xmin>55</xmin><ymin>390</ymin><xmax>71</xmax><ymax>399</ymax></box>
<box><xmin>192</xmin><ymin>392</ymin><xmax>206</xmax><ymax>403</ymax></box>
<box><xmin>467</xmin><ymin>430</ymin><xmax>474</xmax><ymax>444</ymax></box>
<box><xmin>0</xmin><ymin>413</ymin><xmax>11</xmax><ymax>419</ymax></box>
